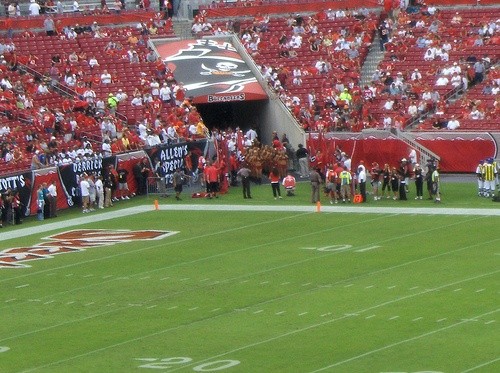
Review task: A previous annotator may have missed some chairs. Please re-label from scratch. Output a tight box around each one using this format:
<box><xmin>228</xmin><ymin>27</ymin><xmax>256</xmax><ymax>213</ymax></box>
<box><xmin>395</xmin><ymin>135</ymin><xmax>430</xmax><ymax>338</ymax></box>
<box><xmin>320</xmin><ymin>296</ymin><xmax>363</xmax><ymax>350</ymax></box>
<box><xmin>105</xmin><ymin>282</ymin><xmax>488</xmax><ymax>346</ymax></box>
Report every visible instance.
<box><xmin>0</xmin><ymin>0</ymin><xmax>500</xmax><ymax>177</ymax></box>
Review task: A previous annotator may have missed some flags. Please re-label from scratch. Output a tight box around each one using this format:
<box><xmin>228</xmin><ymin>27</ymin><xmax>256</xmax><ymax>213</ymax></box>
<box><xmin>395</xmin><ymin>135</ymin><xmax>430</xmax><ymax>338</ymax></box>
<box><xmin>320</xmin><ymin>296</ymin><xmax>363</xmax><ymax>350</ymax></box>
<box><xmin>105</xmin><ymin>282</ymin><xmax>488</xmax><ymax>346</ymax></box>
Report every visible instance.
<box><xmin>317</xmin><ymin>133</ymin><xmax>329</xmax><ymax>164</ymax></box>
<box><xmin>309</xmin><ymin>134</ymin><xmax>322</xmax><ymax>169</ymax></box>
<box><xmin>218</xmin><ymin>139</ymin><xmax>228</xmax><ymax>194</ymax></box>
<box><xmin>236</xmin><ymin>129</ymin><xmax>247</xmax><ymax>156</ymax></box>
<box><xmin>328</xmin><ymin>138</ymin><xmax>335</xmax><ymax>165</ymax></box>
<box><xmin>350</xmin><ymin>140</ymin><xmax>361</xmax><ymax>177</ymax></box>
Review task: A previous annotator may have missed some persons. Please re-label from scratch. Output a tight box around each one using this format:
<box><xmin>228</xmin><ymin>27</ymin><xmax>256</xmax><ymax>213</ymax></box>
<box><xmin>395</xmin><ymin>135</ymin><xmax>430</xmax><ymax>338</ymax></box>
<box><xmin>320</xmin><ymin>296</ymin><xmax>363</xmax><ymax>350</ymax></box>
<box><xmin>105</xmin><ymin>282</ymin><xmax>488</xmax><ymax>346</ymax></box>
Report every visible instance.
<box><xmin>0</xmin><ymin>0</ymin><xmax>500</xmax><ymax>227</ymax></box>
